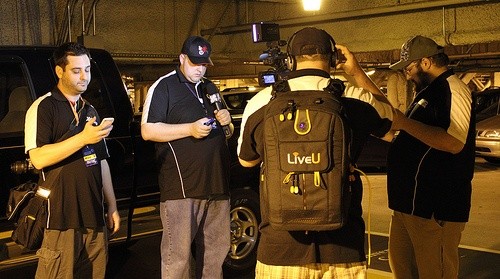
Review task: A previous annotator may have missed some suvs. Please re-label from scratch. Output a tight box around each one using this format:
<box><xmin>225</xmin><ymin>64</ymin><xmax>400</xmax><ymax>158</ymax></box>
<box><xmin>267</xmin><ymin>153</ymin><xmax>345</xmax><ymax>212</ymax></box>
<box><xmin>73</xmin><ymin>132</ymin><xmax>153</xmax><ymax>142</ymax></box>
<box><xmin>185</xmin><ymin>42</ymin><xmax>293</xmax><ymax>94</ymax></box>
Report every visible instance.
<box><xmin>0</xmin><ymin>44</ymin><xmax>500</xmax><ymax>279</ymax></box>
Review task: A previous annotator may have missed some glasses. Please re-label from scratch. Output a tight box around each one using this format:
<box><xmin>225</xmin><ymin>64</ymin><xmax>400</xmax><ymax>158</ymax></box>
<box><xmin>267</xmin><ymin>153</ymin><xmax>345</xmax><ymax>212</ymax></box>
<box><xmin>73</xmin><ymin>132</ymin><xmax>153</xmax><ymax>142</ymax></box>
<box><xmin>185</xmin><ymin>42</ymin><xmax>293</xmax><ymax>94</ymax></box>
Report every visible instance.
<box><xmin>403</xmin><ymin>60</ymin><xmax>420</xmax><ymax>75</ymax></box>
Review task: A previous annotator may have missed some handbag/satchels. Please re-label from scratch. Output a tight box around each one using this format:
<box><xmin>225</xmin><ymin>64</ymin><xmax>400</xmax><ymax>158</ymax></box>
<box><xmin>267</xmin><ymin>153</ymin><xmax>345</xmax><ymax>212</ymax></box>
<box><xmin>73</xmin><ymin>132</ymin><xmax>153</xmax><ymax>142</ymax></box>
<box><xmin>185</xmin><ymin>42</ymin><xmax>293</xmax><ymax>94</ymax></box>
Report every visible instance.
<box><xmin>6</xmin><ymin>181</ymin><xmax>51</xmax><ymax>250</ymax></box>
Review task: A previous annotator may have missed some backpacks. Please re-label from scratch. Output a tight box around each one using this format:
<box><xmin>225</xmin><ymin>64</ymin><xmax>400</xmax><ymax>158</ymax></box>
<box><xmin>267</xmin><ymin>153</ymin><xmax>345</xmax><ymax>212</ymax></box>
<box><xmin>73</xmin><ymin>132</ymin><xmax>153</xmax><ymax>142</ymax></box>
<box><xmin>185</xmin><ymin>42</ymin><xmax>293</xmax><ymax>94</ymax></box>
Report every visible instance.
<box><xmin>263</xmin><ymin>78</ymin><xmax>350</xmax><ymax>231</ymax></box>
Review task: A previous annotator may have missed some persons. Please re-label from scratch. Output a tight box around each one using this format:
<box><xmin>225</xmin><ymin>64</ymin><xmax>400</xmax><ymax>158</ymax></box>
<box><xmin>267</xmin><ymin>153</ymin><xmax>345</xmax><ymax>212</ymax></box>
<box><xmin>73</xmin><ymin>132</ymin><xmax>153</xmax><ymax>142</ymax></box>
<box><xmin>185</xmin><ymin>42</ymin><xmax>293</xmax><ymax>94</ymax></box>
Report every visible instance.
<box><xmin>387</xmin><ymin>34</ymin><xmax>478</xmax><ymax>279</ymax></box>
<box><xmin>237</xmin><ymin>27</ymin><xmax>398</xmax><ymax>279</ymax></box>
<box><xmin>141</xmin><ymin>36</ymin><xmax>234</xmax><ymax>279</ymax></box>
<box><xmin>25</xmin><ymin>42</ymin><xmax>120</xmax><ymax>279</ymax></box>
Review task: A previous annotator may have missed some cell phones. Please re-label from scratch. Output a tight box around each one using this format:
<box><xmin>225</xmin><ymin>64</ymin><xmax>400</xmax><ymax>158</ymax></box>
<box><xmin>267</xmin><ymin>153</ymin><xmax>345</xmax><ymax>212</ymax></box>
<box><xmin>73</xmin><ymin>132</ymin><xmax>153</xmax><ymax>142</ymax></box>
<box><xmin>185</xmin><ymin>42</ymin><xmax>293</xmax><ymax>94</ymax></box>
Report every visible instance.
<box><xmin>101</xmin><ymin>118</ymin><xmax>114</xmax><ymax>127</ymax></box>
<box><xmin>207</xmin><ymin>118</ymin><xmax>216</xmax><ymax>126</ymax></box>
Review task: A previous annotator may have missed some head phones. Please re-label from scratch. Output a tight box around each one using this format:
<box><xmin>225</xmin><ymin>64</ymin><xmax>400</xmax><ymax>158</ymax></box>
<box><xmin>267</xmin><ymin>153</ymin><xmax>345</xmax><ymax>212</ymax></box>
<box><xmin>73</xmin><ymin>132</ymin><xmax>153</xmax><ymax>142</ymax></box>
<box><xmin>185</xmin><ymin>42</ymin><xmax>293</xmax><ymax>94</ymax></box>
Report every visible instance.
<box><xmin>285</xmin><ymin>27</ymin><xmax>339</xmax><ymax>71</ymax></box>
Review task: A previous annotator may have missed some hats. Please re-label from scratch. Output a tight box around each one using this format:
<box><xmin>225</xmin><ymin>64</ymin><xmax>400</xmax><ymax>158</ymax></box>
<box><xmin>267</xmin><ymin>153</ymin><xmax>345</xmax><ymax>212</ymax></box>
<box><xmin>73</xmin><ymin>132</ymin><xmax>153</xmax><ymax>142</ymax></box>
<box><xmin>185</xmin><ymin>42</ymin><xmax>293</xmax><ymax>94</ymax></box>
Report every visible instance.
<box><xmin>290</xmin><ymin>28</ymin><xmax>332</xmax><ymax>55</ymax></box>
<box><xmin>388</xmin><ymin>35</ymin><xmax>445</xmax><ymax>70</ymax></box>
<box><xmin>181</xmin><ymin>36</ymin><xmax>214</xmax><ymax>66</ymax></box>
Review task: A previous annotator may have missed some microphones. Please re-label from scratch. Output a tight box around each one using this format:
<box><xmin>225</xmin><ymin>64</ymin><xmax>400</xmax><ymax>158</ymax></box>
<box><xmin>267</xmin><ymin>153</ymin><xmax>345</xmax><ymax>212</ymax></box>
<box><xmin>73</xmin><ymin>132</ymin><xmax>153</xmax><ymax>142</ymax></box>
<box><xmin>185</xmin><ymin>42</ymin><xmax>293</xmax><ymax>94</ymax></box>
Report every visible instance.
<box><xmin>206</xmin><ymin>83</ymin><xmax>232</xmax><ymax>139</ymax></box>
